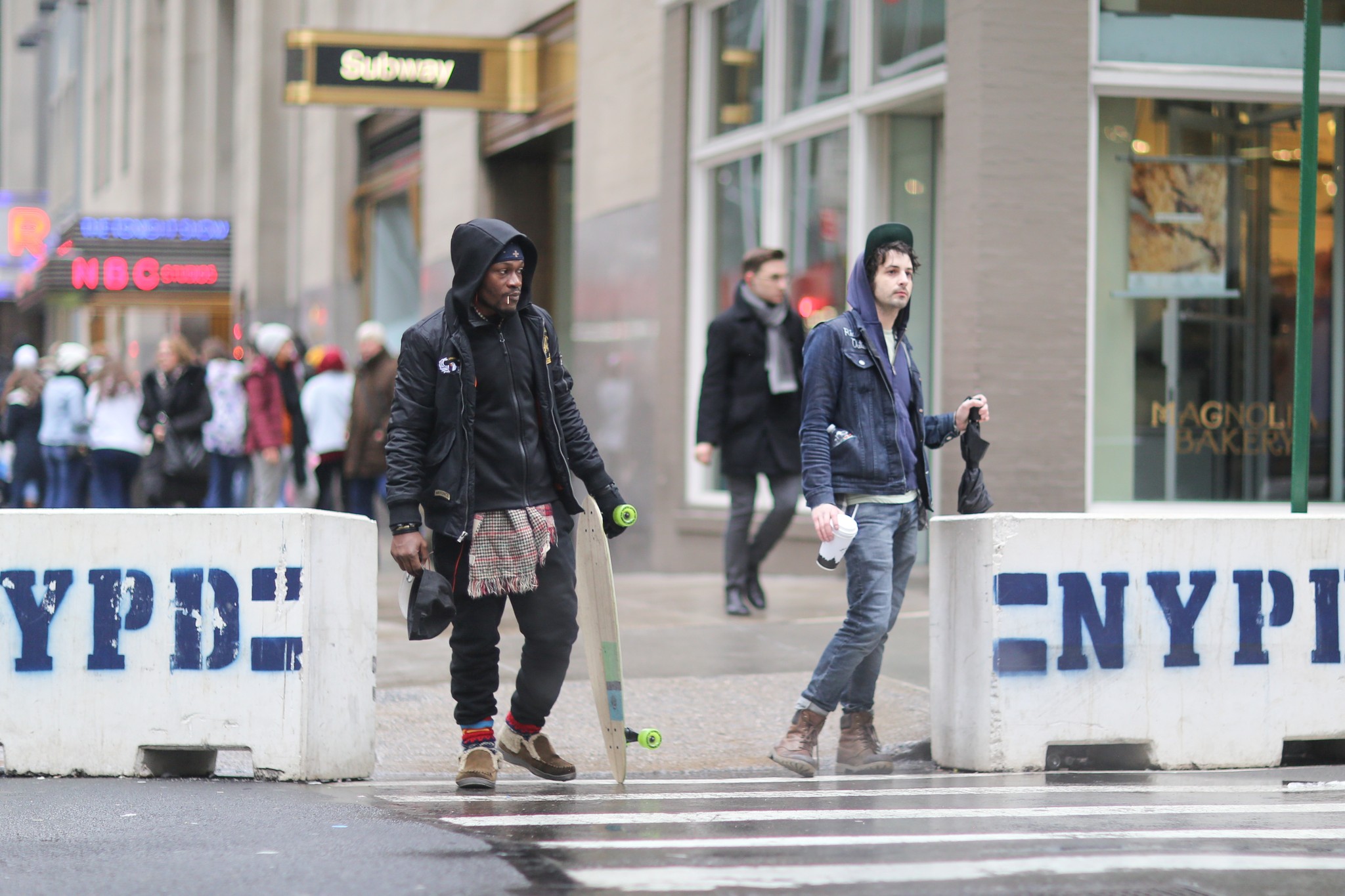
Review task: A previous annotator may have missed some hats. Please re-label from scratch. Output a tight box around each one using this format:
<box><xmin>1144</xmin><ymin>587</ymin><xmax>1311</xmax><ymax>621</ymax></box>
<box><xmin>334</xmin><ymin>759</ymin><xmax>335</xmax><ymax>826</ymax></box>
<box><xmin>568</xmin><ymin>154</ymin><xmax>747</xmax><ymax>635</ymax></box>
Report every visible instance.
<box><xmin>407</xmin><ymin>567</ymin><xmax>456</xmax><ymax>642</ymax></box>
<box><xmin>57</xmin><ymin>343</ymin><xmax>91</xmax><ymax>373</ymax></box>
<box><xmin>249</xmin><ymin>320</ymin><xmax>291</xmax><ymax>360</ymax></box>
<box><xmin>864</xmin><ymin>223</ymin><xmax>914</xmax><ymax>259</ymax></box>
<box><xmin>13</xmin><ymin>345</ymin><xmax>37</xmax><ymax>375</ymax></box>
<box><xmin>306</xmin><ymin>345</ymin><xmax>342</xmax><ymax>371</ymax></box>
<box><xmin>489</xmin><ymin>242</ymin><xmax>524</xmax><ymax>264</ymax></box>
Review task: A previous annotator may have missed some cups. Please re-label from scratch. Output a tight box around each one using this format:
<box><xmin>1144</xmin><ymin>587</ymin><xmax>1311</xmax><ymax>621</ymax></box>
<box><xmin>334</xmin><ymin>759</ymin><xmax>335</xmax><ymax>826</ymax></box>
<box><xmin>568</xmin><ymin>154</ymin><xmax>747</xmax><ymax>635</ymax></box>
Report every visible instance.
<box><xmin>817</xmin><ymin>513</ymin><xmax>857</xmax><ymax>573</ymax></box>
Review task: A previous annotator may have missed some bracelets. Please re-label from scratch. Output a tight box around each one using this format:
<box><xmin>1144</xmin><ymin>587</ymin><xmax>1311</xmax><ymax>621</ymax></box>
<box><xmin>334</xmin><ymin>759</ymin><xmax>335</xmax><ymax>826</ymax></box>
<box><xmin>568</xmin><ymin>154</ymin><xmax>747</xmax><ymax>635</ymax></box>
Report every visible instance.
<box><xmin>392</xmin><ymin>527</ymin><xmax>419</xmax><ymax>535</ymax></box>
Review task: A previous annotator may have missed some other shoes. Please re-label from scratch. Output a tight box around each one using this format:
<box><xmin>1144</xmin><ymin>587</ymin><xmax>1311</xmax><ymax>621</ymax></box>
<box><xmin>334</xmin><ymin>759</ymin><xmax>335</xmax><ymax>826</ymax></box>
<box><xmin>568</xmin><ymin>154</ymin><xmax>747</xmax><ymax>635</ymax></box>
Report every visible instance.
<box><xmin>726</xmin><ymin>586</ymin><xmax>752</xmax><ymax>615</ymax></box>
<box><xmin>454</xmin><ymin>746</ymin><xmax>502</xmax><ymax>789</ymax></box>
<box><xmin>746</xmin><ymin>571</ymin><xmax>763</xmax><ymax>609</ymax></box>
<box><xmin>496</xmin><ymin>722</ymin><xmax>576</xmax><ymax>780</ymax></box>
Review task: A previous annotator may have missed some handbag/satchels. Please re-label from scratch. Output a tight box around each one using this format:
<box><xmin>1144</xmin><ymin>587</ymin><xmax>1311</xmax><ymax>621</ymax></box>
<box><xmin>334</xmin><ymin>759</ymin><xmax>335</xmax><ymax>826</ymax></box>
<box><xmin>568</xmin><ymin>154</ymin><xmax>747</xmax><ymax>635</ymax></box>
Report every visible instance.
<box><xmin>163</xmin><ymin>432</ymin><xmax>208</xmax><ymax>480</ymax></box>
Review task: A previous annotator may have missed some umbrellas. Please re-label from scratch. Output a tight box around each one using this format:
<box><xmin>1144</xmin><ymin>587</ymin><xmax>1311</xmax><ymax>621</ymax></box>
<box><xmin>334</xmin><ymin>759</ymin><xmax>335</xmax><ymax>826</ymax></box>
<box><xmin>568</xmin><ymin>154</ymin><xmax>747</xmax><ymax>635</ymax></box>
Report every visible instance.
<box><xmin>958</xmin><ymin>397</ymin><xmax>994</xmax><ymax>514</ymax></box>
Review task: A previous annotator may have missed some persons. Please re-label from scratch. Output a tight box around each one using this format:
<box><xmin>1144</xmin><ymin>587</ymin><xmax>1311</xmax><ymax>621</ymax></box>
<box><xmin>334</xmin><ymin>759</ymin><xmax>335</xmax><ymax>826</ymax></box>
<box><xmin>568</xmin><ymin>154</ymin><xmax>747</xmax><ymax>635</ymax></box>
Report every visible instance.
<box><xmin>385</xmin><ymin>218</ymin><xmax>626</xmax><ymax>789</ymax></box>
<box><xmin>341</xmin><ymin>320</ymin><xmax>399</xmax><ymax>521</ymax></box>
<box><xmin>299</xmin><ymin>350</ymin><xmax>357</xmax><ymax>513</ymax></box>
<box><xmin>768</xmin><ymin>223</ymin><xmax>990</xmax><ymax>777</ymax></box>
<box><xmin>241</xmin><ymin>321</ymin><xmax>306</xmax><ymax>508</ymax></box>
<box><xmin>695</xmin><ymin>248</ymin><xmax>807</xmax><ymax>614</ymax></box>
<box><xmin>0</xmin><ymin>333</ymin><xmax>253</xmax><ymax>508</ymax></box>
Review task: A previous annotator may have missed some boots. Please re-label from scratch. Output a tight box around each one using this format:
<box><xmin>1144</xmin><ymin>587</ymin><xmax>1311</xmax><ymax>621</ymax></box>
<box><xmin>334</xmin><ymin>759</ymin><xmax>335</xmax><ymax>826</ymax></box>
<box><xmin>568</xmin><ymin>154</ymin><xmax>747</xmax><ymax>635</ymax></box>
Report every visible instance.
<box><xmin>765</xmin><ymin>708</ymin><xmax>827</xmax><ymax>779</ymax></box>
<box><xmin>833</xmin><ymin>711</ymin><xmax>894</xmax><ymax>775</ymax></box>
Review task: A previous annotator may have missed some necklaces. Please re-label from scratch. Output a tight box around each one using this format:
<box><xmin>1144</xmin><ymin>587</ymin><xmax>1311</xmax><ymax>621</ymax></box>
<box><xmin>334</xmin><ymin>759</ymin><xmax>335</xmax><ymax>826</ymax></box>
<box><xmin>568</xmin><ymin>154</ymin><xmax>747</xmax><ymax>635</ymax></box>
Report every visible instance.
<box><xmin>471</xmin><ymin>303</ymin><xmax>503</xmax><ymax>329</ymax></box>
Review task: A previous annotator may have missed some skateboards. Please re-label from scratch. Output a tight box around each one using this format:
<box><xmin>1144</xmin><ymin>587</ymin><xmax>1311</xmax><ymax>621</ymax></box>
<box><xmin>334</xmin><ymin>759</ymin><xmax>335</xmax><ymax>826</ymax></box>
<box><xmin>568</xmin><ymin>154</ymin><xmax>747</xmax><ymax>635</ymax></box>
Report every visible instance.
<box><xmin>575</xmin><ymin>493</ymin><xmax>662</xmax><ymax>786</ymax></box>
